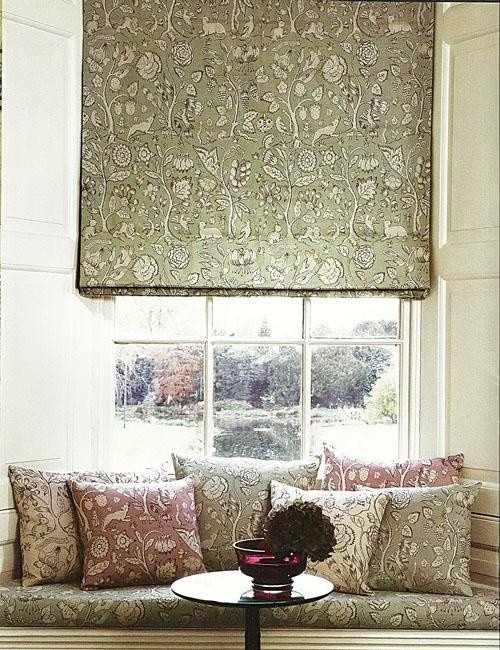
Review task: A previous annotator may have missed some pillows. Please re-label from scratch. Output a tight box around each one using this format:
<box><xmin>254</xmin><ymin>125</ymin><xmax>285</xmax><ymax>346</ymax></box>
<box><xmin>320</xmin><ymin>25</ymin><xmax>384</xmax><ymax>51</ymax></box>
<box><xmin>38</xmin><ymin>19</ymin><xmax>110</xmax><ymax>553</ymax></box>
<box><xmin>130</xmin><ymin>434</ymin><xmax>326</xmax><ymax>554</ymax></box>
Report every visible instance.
<box><xmin>171</xmin><ymin>450</ymin><xmax>323</xmax><ymax>572</ymax></box>
<box><xmin>352</xmin><ymin>480</ymin><xmax>482</xmax><ymax>596</ymax></box>
<box><xmin>66</xmin><ymin>474</ymin><xmax>207</xmax><ymax>591</ymax></box>
<box><xmin>8</xmin><ymin>460</ymin><xmax>169</xmax><ymax>587</ymax></box>
<box><xmin>320</xmin><ymin>438</ymin><xmax>464</xmax><ymax>490</ymax></box>
<box><xmin>260</xmin><ymin>479</ymin><xmax>393</xmax><ymax>596</ymax></box>
